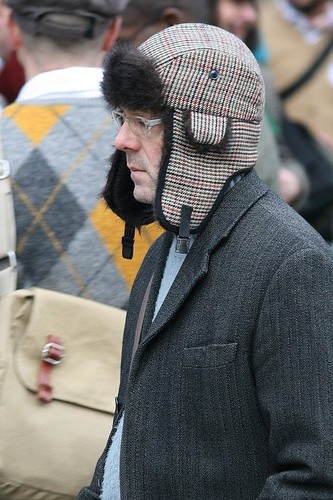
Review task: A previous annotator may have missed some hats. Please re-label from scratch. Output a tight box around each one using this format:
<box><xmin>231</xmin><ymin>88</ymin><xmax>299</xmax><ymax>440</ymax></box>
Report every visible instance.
<box><xmin>5</xmin><ymin>0</ymin><xmax>129</xmax><ymax>41</ymax></box>
<box><xmin>102</xmin><ymin>23</ymin><xmax>266</xmax><ymax>236</ymax></box>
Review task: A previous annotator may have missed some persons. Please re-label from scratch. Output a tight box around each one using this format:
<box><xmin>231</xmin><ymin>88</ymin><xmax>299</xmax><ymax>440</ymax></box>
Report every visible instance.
<box><xmin>0</xmin><ymin>0</ymin><xmax>333</xmax><ymax>311</ymax></box>
<box><xmin>78</xmin><ymin>23</ymin><xmax>333</xmax><ymax>500</ymax></box>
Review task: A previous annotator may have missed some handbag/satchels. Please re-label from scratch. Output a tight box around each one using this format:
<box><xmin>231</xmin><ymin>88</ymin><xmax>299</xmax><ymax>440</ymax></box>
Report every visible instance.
<box><xmin>0</xmin><ymin>288</ymin><xmax>127</xmax><ymax>496</ymax></box>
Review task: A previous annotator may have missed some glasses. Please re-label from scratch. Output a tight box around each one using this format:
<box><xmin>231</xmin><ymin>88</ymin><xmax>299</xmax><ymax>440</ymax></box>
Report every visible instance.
<box><xmin>111</xmin><ymin>107</ymin><xmax>164</xmax><ymax>137</ymax></box>
